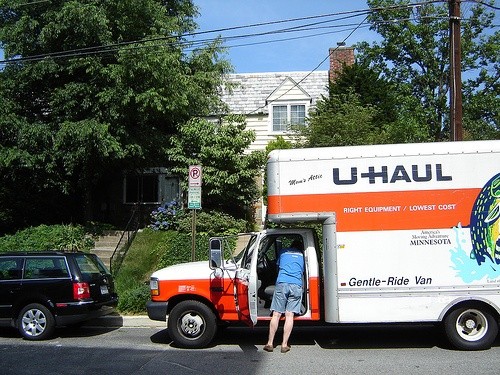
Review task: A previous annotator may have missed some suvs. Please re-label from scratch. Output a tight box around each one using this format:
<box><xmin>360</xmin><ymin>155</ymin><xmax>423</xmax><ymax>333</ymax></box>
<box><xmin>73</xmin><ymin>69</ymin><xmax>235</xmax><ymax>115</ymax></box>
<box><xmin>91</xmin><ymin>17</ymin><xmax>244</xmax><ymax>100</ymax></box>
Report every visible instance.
<box><xmin>0</xmin><ymin>250</ymin><xmax>118</xmax><ymax>341</ymax></box>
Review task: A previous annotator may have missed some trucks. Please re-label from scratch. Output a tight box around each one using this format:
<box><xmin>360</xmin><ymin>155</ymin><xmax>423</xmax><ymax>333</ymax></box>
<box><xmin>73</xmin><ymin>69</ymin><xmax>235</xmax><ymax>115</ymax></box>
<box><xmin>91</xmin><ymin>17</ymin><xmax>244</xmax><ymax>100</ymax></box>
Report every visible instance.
<box><xmin>146</xmin><ymin>139</ymin><xmax>499</xmax><ymax>350</ymax></box>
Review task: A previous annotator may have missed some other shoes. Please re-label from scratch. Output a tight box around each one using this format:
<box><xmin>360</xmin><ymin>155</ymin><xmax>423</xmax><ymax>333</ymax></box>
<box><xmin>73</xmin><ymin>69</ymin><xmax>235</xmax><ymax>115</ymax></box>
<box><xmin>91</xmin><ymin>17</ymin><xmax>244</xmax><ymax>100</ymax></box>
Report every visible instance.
<box><xmin>263</xmin><ymin>345</ymin><xmax>273</xmax><ymax>352</ymax></box>
<box><xmin>281</xmin><ymin>346</ymin><xmax>290</xmax><ymax>353</ymax></box>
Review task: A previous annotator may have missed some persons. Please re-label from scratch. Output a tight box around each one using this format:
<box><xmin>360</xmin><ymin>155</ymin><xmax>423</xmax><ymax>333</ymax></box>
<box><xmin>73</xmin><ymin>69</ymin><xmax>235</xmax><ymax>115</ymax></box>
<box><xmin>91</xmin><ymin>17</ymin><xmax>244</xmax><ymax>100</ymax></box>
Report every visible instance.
<box><xmin>262</xmin><ymin>240</ymin><xmax>304</xmax><ymax>353</ymax></box>
